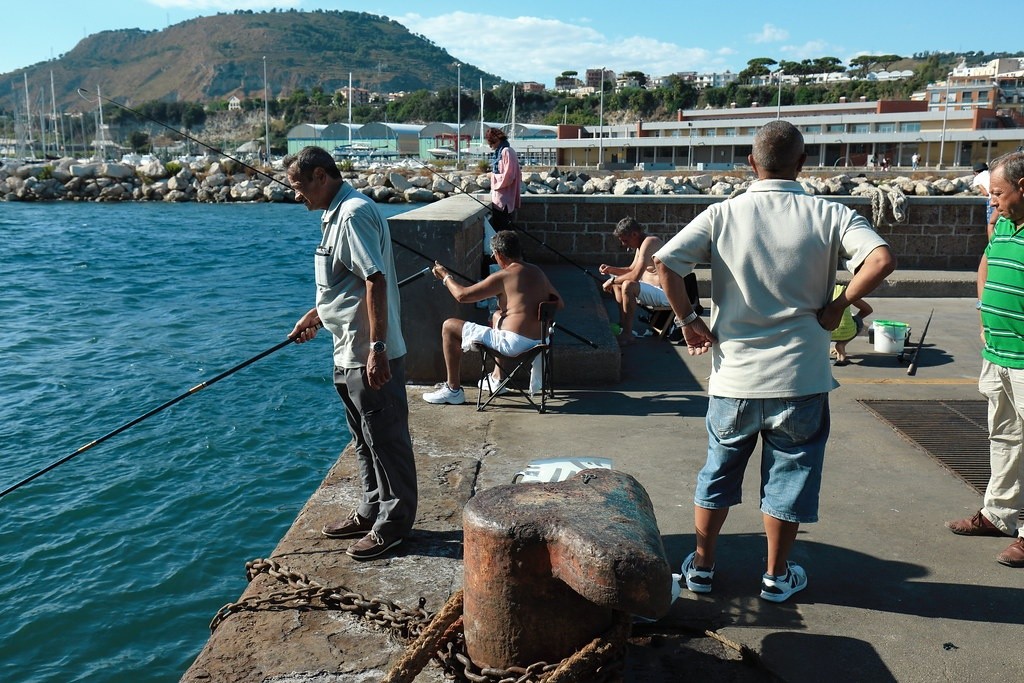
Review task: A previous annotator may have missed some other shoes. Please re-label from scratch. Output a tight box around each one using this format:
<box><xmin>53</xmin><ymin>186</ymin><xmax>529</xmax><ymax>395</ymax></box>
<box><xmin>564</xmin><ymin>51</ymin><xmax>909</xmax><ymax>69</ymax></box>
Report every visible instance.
<box><xmin>617</xmin><ymin>335</ymin><xmax>636</xmax><ymax>346</ymax></box>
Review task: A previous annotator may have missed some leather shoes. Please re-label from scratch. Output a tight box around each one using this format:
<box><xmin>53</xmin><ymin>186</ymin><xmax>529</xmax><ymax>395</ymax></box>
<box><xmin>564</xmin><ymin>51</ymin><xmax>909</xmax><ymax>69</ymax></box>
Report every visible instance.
<box><xmin>346</xmin><ymin>528</ymin><xmax>403</xmax><ymax>558</ymax></box>
<box><xmin>997</xmin><ymin>536</ymin><xmax>1024</xmax><ymax>566</ymax></box>
<box><xmin>321</xmin><ymin>509</ymin><xmax>375</xmax><ymax>536</ymax></box>
<box><xmin>945</xmin><ymin>507</ymin><xmax>1019</xmax><ymax>537</ymax></box>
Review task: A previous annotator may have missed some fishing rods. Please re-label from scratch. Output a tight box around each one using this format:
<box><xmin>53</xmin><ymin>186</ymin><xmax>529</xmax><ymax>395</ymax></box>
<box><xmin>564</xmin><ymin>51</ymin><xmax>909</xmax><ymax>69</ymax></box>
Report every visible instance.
<box><xmin>0</xmin><ymin>265</ymin><xmax>433</xmax><ymax>498</ymax></box>
<box><xmin>75</xmin><ymin>86</ymin><xmax>601</xmax><ymax>351</ymax></box>
<box><xmin>376</xmin><ymin>135</ymin><xmax>653</xmax><ymax>323</ymax></box>
<box><xmin>906</xmin><ymin>307</ymin><xmax>934</xmax><ymax>376</ymax></box>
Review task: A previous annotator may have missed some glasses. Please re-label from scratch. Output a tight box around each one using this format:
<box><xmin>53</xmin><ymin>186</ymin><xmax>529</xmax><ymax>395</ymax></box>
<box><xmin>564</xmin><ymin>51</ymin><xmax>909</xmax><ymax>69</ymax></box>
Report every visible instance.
<box><xmin>490</xmin><ymin>249</ymin><xmax>501</xmax><ymax>262</ymax></box>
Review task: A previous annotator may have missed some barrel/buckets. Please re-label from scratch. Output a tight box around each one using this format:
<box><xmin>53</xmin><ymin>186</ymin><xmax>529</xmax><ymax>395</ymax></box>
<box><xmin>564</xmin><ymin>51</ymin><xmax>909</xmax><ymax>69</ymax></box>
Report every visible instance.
<box><xmin>873</xmin><ymin>319</ymin><xmax>907</xmax><ymax>353</ymax></box>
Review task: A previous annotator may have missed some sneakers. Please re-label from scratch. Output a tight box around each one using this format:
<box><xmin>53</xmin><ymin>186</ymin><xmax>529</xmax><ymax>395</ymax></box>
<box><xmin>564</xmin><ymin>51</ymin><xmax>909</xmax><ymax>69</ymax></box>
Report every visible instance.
<box><xmin>761</xmin><ymin>562</ymin><xmax>807</xmax><ymax>602</ymax></box>
<box><xmin>681</xmin><ymin>549</ymin><xmax>717</xmax><ymax>592</ymax></box>
<box><xmin>423</xmin><ymin>382</ymin><xmax>464</xmax><ymax>404</ymax></box>
<box><xmin>478</xmin><ymin>371</ymin><xmax>507</xmax><ymax>393</ymax></box>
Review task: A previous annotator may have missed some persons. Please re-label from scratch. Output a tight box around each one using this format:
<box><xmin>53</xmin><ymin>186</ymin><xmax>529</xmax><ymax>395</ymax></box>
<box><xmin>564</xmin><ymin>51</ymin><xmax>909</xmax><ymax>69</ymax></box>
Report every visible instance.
<box><xmin>945</xmin><ymin>151</ymin><xmax>1024</xmax><ymax>565</ymax></box>
<box><xmin>972</xmin><ymin>161</ymin><xmax>999</xmax><ymax>240</ymax></box>
<box><xmin>651</xmin><ymin>120</ymin><xmax>897</xmax><ymax>602</ymax></box>
<box><xmin>830</xmin><ymin>284</ymin><xmax>873</xmax><ymax>367</ymax></box>
<box><xmin>872</xmin><ymin>152</ymin><xmax>922</xmax><ymax>171</ymax></box>
<box><xmin>422</xmin><ymin>230</ymin><xmax>564</xmax><ymax>404</ymax></box>
<box><xmin>282</xmin><ymin>146</ymin><xmax>419</xmax><ymax>558</ymax></box>
<box><xmin>484</xmin><ymin>128</ymin><xmax>521</xmax><ymax>231</ymax></box>
<box><xmin>258</xmin><ymin>145</ymin><xmax>266</xmax><ymax>167</ymax></box>
<box><xmin>599</xmin><ymin>217</ymin><xmax>671</xmax><ymax>343</ymax></box>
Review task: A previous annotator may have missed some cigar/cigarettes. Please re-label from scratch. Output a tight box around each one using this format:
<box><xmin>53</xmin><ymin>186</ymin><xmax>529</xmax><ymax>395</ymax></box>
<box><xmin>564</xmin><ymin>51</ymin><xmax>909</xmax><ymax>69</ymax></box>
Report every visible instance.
<box><xmin>627</xmin><ymin>245</ymin><xmax>629</xmax><ymax>251</ymax></box>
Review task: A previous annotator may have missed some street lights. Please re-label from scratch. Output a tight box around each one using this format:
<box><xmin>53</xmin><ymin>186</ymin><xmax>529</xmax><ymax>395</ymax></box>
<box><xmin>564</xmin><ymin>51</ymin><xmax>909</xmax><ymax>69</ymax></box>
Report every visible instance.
<box><xmin>263</xmin><ymin>56</ymin><xmax>269</xmax><ymax>156</ymax></box>
<box><xmin>776</xmin><ymin>69</ymin><xmax>784</xmax><ymax>120</ymax></box>
<box><xmin>936</xmin><ymin>71</ymin><xmax>953</xmax><ymax>169</ymax></box>
<box><xmin>598</xmin><ymin>67</ymin><xmax>605</xmax><ymax>163</ymax></box>
<box><xmin>457</xmin><ymin>63</ymin><xmax>461</xmax><ymax>162</ymax></box>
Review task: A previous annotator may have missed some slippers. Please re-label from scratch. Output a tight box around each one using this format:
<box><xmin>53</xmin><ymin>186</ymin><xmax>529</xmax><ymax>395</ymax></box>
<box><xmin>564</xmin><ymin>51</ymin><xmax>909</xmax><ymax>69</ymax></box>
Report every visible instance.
<box><xmin>834</xmin><ymin>355</ymin><xmax>849</xmax><ymax>366</ymax></box>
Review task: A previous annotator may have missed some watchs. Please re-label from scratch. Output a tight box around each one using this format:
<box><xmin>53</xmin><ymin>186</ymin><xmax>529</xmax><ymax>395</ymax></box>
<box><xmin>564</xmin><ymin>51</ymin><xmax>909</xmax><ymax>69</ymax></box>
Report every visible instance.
<box><xmin>370</xmin><ymin>341</ymin><xmax>387</xmax><ymax>353</ymax></box>
<box><xmin>975</xmin><ymin>300</ymin><xmax>981</xmax><ymax>310</ymax></box>
<box><xmin>611</xmin><ymin>277</ymin><xmax>615</xmax><ymax>284</ymax></box>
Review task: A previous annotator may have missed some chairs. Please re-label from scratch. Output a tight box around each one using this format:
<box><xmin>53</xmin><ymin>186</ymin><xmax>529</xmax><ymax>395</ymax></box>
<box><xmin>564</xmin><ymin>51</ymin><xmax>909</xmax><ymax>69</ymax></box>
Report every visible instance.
<box><xmin>474</xmin><ymin>294</ymin><xmax>559</xmax><ymax>415</ymax></box>
<box><xmin>637</xmin><ymin>273</ymin><xmax>702</xmax><ymax>343</ymax></box>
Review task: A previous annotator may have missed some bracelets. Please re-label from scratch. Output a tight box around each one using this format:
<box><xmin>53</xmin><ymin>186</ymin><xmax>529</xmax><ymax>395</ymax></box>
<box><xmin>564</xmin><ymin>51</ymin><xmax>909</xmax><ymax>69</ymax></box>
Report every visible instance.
<box><xmin>442</xmin><ymin>274</ymin><xmax>453</xmax><ymax>286</ymax></box>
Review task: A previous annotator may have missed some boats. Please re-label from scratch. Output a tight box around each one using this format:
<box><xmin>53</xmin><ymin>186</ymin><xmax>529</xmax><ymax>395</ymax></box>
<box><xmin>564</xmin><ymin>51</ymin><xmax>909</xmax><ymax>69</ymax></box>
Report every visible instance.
<box><xmin>427</xmin><ymin>144</ymin><xmax>457</xmax><ymax>158</ymax></box>
<box><xmin>334</xmin><ymin>144</ymin><xmax>418</xmax><ymax>157</ymax></box>
<box><xmin>460</xmin><ymin>144</ymin><xmax>493</xmax><ymax>154</ymax></box>
